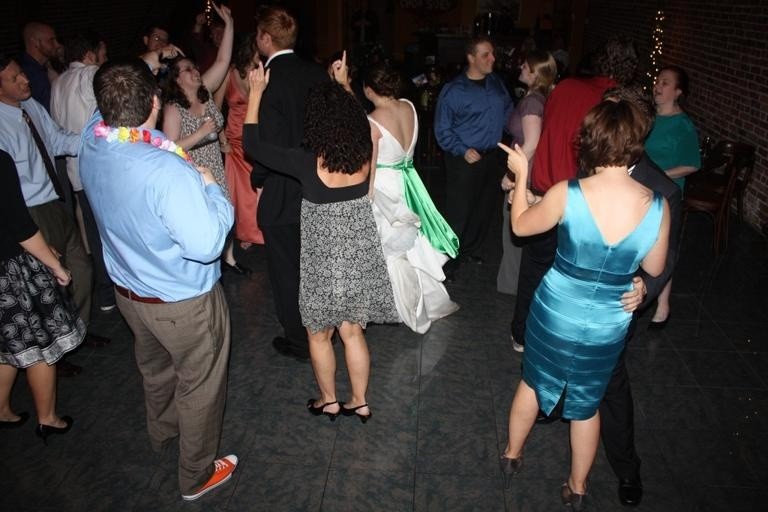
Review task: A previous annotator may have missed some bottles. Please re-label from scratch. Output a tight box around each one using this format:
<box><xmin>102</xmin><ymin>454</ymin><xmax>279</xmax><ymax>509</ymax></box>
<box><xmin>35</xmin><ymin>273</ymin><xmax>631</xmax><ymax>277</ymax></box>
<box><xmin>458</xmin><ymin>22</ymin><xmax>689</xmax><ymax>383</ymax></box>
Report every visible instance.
<box><xmin>702</xmin><ymin>135</ymin><xmax>711</xmax><ymax>163</ymax></box>
<box><xmin>204</xmin><ymin>110</ymin><xmax>217</xmax><ymax>142</ymax></box>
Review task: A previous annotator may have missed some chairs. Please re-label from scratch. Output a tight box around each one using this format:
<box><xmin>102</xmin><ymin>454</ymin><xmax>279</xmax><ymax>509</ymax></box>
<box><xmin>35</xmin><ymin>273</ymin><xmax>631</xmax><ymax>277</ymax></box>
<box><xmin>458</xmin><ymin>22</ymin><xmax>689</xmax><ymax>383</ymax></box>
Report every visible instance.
<box><xmin>692</xmin><ymin>139</ymin><xmax>756</xmax><ymax>239</ymax></box>
<box><xmin>675</xmin><ymin>158</ymin><xmax>736</xmax><ymax>264</ymax></box>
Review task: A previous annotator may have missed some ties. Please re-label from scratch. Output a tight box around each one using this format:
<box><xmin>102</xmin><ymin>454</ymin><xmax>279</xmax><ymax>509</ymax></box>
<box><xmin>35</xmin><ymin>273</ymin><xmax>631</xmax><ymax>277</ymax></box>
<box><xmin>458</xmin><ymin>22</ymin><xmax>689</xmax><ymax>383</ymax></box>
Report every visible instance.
<box><xmin>22</xmin><ymin>108</ymin><xmax>66</xmax><ymax>202</ymax></box>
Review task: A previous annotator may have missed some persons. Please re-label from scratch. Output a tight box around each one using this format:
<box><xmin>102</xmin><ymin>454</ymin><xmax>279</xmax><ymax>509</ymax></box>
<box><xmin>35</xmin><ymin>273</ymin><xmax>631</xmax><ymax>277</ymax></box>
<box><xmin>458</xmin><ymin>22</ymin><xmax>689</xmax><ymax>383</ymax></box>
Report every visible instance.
<box><xmin>162</xmin><ymin>2</ymin><xmax>244</xmax><ymax>279</ymax></box>
<box><xmin>363</xmin><ymin>64</ymin><xmax>459</xmax><ymax>334</ymax></box>
<box><xmin>0</xmin><ymin>58</ymin><xmax>91</xmax><ymax>381</ymax></box>
<box><xmin>79</xmin><ymin>51</ymin><xmax>239</xmax><ymax>501</ymax></box>
<box><xmin>435</xmin><ymin>40</ymin><xmax>515</xmax><ymax>283</ymax></box>
<box><xmin>498</xmin><ymin>99</ymin><xmax>669</xmax><ymax>512</ymax></box>
<box><xmin>645</xmin><ymin>69</ymin><xmax>702</xmax><ymax>331</ymax></box>
<box><xmin>243</xmin><ymin>51</ymin><xmax>399</xmax><ymax>424</ymax></box>
<box><xmin>249</xmin><ymin>12</ymin><xmax>328</xmax><ymax>364</ymax></box>
<box><xmin>50</xmin><ymin>30</ymin><xmax>116</xmax><ymax>311</ymax></box>
<box><xmin>18</xmin><ymin>22</ymin><xmax>61</xmax><ymax>114</ymax></box>
<box><xmin>0</xmin><ymin>150</ymin><xmax>86</xmax><ymax>444</ymax></box>
<box><xmin>510</xmin><ymin>41</ymin><xmax>648</xmax><ymax>351</ymax></box>
<box><xmin>508</xmin><ymin>84</ymin><xmax>683</xmax><ymax>509</ymax></box>
<box><xmin>497</xmin><ymin>49</ymin><xmax>567</xmax><ymax>296</ymax></box>
<box><xmin>144</xmin><ymin>26</ymin><xmax>168</xmax><ymax>51</ymax></box>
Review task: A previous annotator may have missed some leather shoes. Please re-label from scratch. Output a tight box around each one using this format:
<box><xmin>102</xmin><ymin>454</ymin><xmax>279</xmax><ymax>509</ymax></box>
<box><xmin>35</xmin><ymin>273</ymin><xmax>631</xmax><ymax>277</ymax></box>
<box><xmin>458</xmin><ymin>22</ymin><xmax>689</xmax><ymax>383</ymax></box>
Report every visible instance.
<box><xmin>619</xmin><ymin>474</ymin><xmax>643</xmax><ymax>505</ymax></box>
<box><xmin>536</xmin><ymin>407</ymin><xmax>562</xmax><ymax>424</ymax></box>
<box><xmin>57</xmin><ymin>360</ymin><xmax>81</xmax><ymax>377</ymax></box>
<box><xmin>272</xmin><ymin>337</ymin><xmax>311</xmax><ymax>363</ymax></box>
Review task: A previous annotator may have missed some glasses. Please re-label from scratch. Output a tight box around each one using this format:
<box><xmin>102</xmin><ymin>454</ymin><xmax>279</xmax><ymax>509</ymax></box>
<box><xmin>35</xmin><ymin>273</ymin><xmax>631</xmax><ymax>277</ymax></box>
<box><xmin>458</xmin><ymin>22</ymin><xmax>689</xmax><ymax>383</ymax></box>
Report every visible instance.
<box><xmin>179</xmin><ymin>63</ymin><xmax>199</xmax><ymax>73</ymax></box>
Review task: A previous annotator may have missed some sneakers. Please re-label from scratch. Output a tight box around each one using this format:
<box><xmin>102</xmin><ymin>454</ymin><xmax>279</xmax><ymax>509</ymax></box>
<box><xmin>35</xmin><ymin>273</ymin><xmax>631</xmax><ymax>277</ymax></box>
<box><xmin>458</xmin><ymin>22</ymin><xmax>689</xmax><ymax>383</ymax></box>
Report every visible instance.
<box><xmin>181</xmin><ymin>453</ymin><xmax>238</xmax><ymax>502</ymax></box>
<box><xmin>510</xmin><ymin>332</ymin><xmax>525</xmax><ymax>352</ymax></box>
<box><xmin>99</xmin><ymin>305</ymin><xmax>116</xmax><ymax>310</ymax></box>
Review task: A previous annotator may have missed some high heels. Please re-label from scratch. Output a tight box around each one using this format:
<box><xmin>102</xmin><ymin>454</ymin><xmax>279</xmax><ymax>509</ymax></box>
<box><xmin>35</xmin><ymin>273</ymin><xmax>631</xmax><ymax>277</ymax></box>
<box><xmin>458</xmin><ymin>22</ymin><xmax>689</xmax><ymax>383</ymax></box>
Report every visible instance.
<box><xmin>646</xmin><ymin>307</ymin><xmax>671</xmax><ymax>330</ymax></box>
<box><xmin>338</xmin><ymin>401</ymin><xmax>372</xmax><ymax>424</ymax></box>
<box><xmin>222</xmin><ymin>258</ymin><xmax>251</xmax><ymax>274</ymax></box>
<box><xmin>307</xmin><ymin>398</ymin><xmax>341</xmax><ymax>422</ymax></box>
<box><xmin>499</xmin><ymin>450</ymin><xmax>523</xmax><ymax>489</ymax></box>
<box><xmin>558</xmin><ymin>481</ymin><xmax>588</xmax><ymax>511</ymax></box>
<box><xmin>36</xmin><ymin>414</ymin><xmax>73</xmax><ymax>445</ymax></box>
<box><xmin>0</xmin><ymin>411</ymin><xmax>28</xmax><ymax>428</ymax></box>
